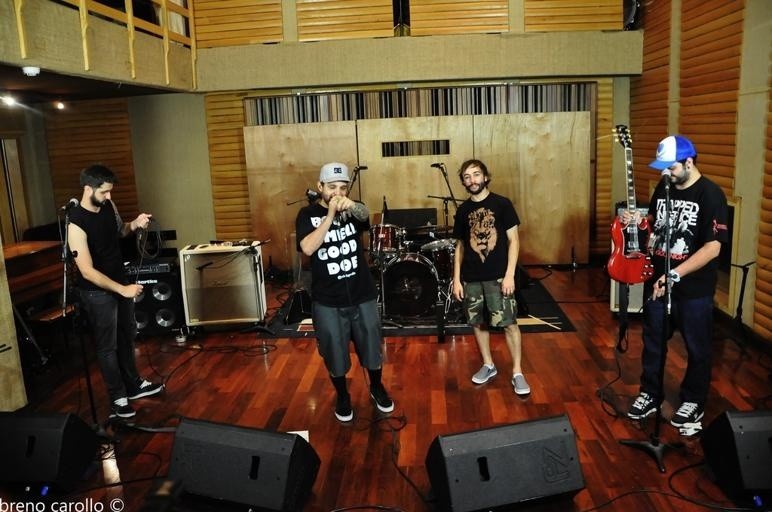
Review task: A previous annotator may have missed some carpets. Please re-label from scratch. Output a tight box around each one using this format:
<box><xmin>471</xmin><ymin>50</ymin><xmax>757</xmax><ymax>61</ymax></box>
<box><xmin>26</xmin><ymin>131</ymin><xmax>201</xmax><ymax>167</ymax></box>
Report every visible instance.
<box><xmin>256</xmin><ymin>278</ymin><xmax>577</xmax><ymax>340</ymax></box>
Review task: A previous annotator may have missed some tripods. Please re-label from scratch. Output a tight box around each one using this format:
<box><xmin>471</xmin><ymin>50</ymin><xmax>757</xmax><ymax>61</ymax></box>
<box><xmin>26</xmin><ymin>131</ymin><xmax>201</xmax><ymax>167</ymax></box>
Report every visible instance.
<box><xmin>240</xmin><ymin>249</ymin><xmax>276</xmax><ymax>335</ymax></box>
<box><xmin>713</xmin><ymin>262</ymin><xmax>756</xmax><ymax>364</ymax></box>
<box><xmin>618</xmin><ymin>281</ymin><xmax>686</xmax><ymax>473</ymax></box>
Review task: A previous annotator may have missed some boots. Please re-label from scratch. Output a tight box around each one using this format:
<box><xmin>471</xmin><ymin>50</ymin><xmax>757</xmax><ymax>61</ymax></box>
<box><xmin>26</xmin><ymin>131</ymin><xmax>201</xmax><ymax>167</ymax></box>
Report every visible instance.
<box><xmin>367</xmin><ymin>366</ymin><xmax>394</xmax><ymax>413</ymax></box>
<box><xmin>329</xmin><ymin>374</ymin><xmax>353</xmax><ymax>421</ymax></box>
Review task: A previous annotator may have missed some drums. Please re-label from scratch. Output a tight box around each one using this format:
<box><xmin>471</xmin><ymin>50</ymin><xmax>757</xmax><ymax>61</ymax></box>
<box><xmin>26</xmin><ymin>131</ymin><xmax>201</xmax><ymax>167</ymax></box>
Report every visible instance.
<box><xmin>368</xmin><ymin>224</ymin><xmax>407</xmax><ymax>252</ymax></box>
<box><xmin>421</xmin><ymin>238</ymin><xmax>457</xmax><ymax>280</ymax></box>
<box><xmin>376</xmin><ymin>252</ymin><xmax>441</xmax><ymax>318</ymax></box>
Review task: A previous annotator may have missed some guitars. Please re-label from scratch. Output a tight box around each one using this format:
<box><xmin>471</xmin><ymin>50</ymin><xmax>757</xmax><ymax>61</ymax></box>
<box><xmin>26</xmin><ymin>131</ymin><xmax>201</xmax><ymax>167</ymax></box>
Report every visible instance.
<box><xmin>607</xmin><ymin>125</ymin><xmax>654</xmax><ymax>284</ymax></box>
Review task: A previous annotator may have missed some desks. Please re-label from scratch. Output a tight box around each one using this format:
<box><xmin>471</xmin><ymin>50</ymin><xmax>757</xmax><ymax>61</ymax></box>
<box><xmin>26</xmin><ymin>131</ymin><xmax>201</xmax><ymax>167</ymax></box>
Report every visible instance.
<box><xmin>3</xmin><ymin>239</ymin><xmax>76</xmax><ymax>338</ymax></box>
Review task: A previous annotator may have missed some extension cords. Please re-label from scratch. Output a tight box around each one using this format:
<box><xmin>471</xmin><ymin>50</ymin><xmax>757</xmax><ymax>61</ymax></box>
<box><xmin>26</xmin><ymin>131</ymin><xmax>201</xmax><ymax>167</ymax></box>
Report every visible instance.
<box><xmin>175</xmin><ymin>334</ymin><xmax>187</xmax><ymax>343</ymax></box>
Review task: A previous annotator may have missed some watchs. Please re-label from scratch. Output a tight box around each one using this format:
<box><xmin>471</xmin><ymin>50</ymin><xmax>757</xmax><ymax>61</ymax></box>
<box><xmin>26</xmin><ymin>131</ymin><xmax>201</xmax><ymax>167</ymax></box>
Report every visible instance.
<box><xmin>670</xmin><ymin>269</ymin><xmax>680</xmax><ymax>283</ymax></box>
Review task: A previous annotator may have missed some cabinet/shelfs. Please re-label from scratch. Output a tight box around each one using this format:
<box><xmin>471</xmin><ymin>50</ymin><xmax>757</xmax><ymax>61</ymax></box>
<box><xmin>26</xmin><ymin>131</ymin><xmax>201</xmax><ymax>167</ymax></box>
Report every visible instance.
<box><xmin>179</xmin><ymin>240</ymin><xmax>267</xmax><ymax>327</ymax></box>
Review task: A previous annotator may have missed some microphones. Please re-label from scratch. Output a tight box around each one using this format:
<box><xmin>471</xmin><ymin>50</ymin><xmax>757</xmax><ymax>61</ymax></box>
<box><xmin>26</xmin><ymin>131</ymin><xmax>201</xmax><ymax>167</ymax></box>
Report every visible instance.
<box><xmin>58</xmin><ymin>197</ymin><xmax>80</xmax><ymax>212</ymax></box>
<box><xmin>431</xmin><ymin>164</ymin><xmax>441</xmax><ymax>167</ymax></box>
<box><xmin>356</xmin><ymin>166</ymin><xmax>367</xmax><ymax>169</ymax></box>
<box><xmin>661</xmin><ymin>169</ymin><xmax>672</xmax><ymax>188</ymax></box>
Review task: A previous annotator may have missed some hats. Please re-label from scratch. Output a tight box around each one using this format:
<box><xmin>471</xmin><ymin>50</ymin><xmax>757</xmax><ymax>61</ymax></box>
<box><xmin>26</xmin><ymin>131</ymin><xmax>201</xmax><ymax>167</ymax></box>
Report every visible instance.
<box><xmin>319</xmin><ymin>163</ymin><xmax>350</xmax><ymax>183</ymax></box>
<box><xmin>648</xmin><ymin>135</ymin><xmax>697</xmax><ymax>171</ymax></box>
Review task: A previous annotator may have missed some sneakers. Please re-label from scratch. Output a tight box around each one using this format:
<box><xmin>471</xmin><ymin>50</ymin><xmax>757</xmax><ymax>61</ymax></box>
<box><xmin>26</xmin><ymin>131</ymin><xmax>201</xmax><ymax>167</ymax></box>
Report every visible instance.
<box><xmin>472</xmin><ymin>364</ymin><xmax>498</xmax><ymax>385</ymax></box>
<box><xmin>627</xmin><ymin>392</ymin><xmax>658</xmax><ymax>419</ymax></box>
<box><xmin>670</xmin><ymin>402</ymin><xmax>704</xmax><ymax>428</ymax></box>
<box><xmin>512</xmin><ymin>373</ymin><xmax>530</xmax><ymax>395</ymax></box>
<box><xmin>128</xmin><ymin>379</ymin><xmax>166</xmax><ymax>400</ymax></box>
<box><xmin>112</xmin><ymin>397</ymin><xmax>136</xmax><ymax>418</ymax></box>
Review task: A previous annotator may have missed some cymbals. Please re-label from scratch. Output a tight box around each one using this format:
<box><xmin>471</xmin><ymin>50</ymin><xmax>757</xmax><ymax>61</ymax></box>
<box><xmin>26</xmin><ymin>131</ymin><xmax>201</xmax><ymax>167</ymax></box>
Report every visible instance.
<box><xmin>413</xmin><ymin>224</ymin><xmax>453</xmax><ymax>234</ymax></box>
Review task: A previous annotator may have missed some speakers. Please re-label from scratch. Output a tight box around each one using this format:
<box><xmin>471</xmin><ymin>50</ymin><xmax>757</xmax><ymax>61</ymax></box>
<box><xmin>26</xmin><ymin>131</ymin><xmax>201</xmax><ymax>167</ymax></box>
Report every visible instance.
<box><xmin>127</xmin><ymin>265</ymin><xmax>186</xmax><ymax>338</ymax></box>
<box><xmin>425</xmin><ymin>412</ymin><xmax>587</xmax><ymax>512</ymax></box>
<box><xmin>165</xmin><ymin>415</ymin><xmax>321</xmax><ymax>512</ymax></box>
<box><xmin>0</xmin><ymin>411</ymin><xmax>100</xmax><ymax>512</ymax></box>
<box><xmin>699</xmin><ymin>410</ymin><xmax>772</xmax><ymax>512</ymax></box>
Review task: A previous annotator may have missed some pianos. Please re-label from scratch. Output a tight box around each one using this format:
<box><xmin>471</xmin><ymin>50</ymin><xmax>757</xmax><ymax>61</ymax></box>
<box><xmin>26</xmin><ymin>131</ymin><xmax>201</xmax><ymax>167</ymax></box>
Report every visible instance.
<box><xmin>2</xmin><ymin>240</ymin><xmax>74</xmax><ymax>364</ymax></box>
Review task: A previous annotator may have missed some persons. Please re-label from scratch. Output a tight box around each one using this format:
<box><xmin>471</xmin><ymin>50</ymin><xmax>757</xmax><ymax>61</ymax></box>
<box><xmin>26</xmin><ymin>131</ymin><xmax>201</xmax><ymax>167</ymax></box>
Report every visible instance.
<box><xmin>295</xmin><ymin>161</ymin><xmax>395</xmax><ymax>423</ymax></box>
<box><xmin>67</xmin><ymin>165</ymin><xmax>166</xmax><ymax>418</ymax></box>
<box><xmin>622</xmin><ymin>136</ymin><xmax>730</xmax><ymax>429</ymax></box>
<box><xmin>453</xmin><ymin>160</ymin><xmax>531</xmax><ymax>396</ymax></box>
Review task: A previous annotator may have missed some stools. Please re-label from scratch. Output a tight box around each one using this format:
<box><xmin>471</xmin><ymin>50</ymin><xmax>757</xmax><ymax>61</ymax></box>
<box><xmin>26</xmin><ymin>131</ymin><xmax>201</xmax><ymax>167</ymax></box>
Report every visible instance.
<box><xmin>26</xmin><ymin>304</ymin><xmax>86</xmax><ymax>360</ymax></box>
